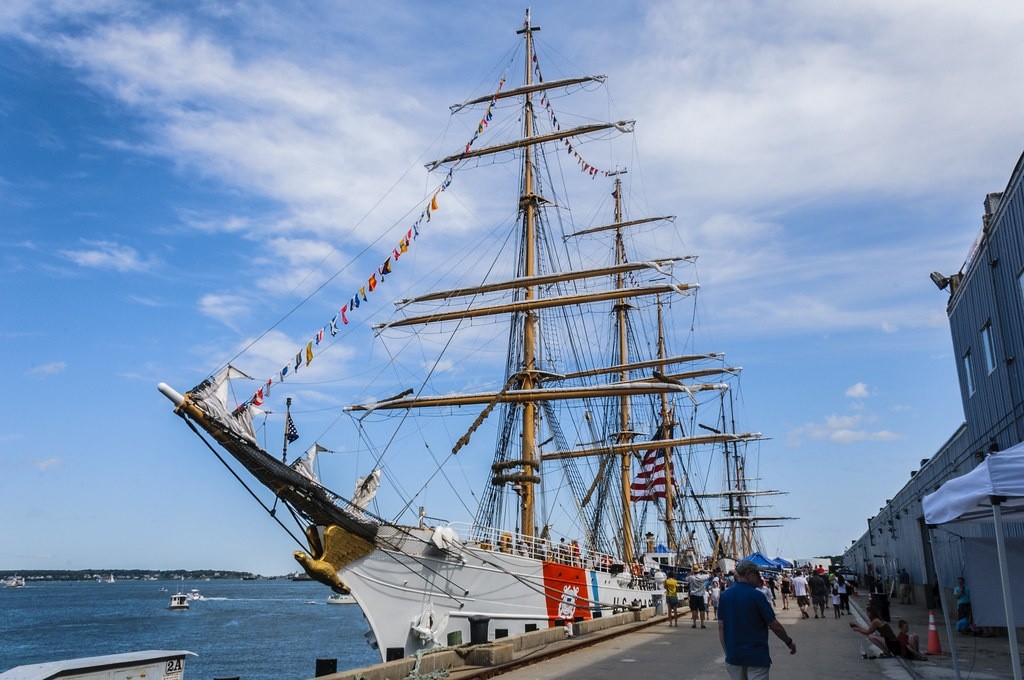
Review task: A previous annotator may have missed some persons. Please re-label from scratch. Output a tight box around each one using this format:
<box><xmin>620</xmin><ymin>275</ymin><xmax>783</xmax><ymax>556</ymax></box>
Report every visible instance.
<box><xmin>718</xmin><ymin>559</ymin><xmax>796</xmax><ymax>680</ymax></box>
<box><xmin>852</xmin><ymin>606</ymin><xmax>928</xmax><ymax>661</ymax></box>
<box><xmin>519</xmin><ymin>539</ymin><xmax>529</xmax><ymax>557</ymax></box>
<box><xmin>898</xmin><ymin>568</ymin><xmax>912</xmax><ymax>604</ymax></box>
<box><xmin>953</xmin><ymin>577</ymin><xmax>993</xmax><ymax>637</ymax></box>
<box><xmin>540</xmin><ymin>538</ymin><xmax>580</xmax><ymax>568</ymax></box>
<box><xmin>663</xmin><ymin>571</ymin><xmax>678</xmax><ymax>627</ymax></box>
<box><xmin>684</xmin><ymin>564</ymin><xmax>735</xmax><ymax>629</ymax></box>
<box><xmin>755</xmin><ymin>561</ymin><xmax>853</xmax><ymax>619</ymax></box>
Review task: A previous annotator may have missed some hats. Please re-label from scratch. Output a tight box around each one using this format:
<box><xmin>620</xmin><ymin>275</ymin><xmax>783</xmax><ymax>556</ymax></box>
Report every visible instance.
<box><xmin>796</xmin><ymin>571</ymin><xmax>802</xmax><ymax>576</ymax></box>
<box><xmin>691</xmin><ymin>564</ymin><xmax>701</xmax><ymax>572</ymax></box>
<box><xmin>736</xmin><ymin>559</ymin><xmax>769</xmax><ymax>574</ymax></box>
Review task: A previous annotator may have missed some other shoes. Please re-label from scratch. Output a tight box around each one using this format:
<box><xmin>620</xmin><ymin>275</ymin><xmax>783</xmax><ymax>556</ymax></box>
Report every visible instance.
<box><xmin>814</xmin><ymin>614</ymin><xmax>818</xmax><ymax>618</ymax></box>
<box><xmin>821</xmin><ymin>615</ymin><xmax>825</xmax><ymax>617</ymax></box>
<box><xmin>692</xmin><ymin>625</ymin><xmax>696</xmax><ymax>628</ymax></box>
<box><xmin>701</xmin><ymin>626</ymin><xmax>705</xmax><ymax>628</ymax></box>
<box><xmin>802</xmin><ymin>617</ymin><xmax>804</xmax><ymax>619</ymax></box>
<box><xmin>804</xmin><ymin>611</ymin><xmax>809</xmax><ymax>619</ymax></box>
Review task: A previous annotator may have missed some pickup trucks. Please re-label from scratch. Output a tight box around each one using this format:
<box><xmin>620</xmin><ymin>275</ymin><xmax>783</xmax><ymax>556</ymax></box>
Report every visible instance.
<box><xmin>838</xmin><ymin>568</ymin><xmax>859</xmax><ymax>584</ymax></box>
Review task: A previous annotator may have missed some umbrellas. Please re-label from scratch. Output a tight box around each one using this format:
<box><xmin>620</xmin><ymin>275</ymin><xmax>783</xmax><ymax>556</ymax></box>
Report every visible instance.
<box><xmin>743</xmin><ymin>551</ymin><xmax>783</xmax><ymax>572</ymax></box>
<box><xmin>772</xmin><ymin>557</ymin><xmax>793</xmax><ymax>569</ymax></box>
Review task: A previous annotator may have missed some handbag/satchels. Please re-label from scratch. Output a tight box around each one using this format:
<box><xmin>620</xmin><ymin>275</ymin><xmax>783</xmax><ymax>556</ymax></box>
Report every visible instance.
<box><xmin>845</xmin><ymin>583</ymin><xmax>855</xmax><ymax>596</ymax></box>
<box><xmin>669</xmin><ymin>596</ymin><xmax>677</xmax><ymax>604</ymax></box>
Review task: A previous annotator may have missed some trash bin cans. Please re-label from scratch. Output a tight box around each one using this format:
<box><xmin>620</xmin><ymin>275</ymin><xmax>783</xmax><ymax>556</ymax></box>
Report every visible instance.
<box><xmin>871</xmin><ymin>593</ymin><xmax>890</xmax><ymax>622</ymax></box>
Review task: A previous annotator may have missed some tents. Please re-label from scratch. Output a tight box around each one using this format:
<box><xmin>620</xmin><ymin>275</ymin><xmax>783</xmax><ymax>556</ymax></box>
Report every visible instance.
<box><xmin>922</xmin><ymin>439</ymin><xmax>1024</xmax><ymax>680</ymax></box>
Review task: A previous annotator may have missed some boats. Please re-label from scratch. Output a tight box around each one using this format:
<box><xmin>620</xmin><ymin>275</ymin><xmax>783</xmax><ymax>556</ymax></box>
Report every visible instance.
<box><xmin>292</xmin><ymin>571</ymin><xmax>314</xmax><ymax>581</ymax></box>
<box><xmin>327</xmin><ymin>592</ymin><xmax>357</xmax><ymax>604</ymax></box>
<box><xmin>140</xmin><ymin>575</ymin><xmax>210</xmax><ymax>581</ymax></box>
<box><xmin>242</xmin><ymin>573</ymin><xmax>277</xmax><ymax>580</ymax></box>
<box><xmin>169</xmin><ymin>594</ymin><xmax>190</xmax><ymax>610</ymax></box>
<box><xmin>188</xmin><ymin>589</ymin><xmax>202</xmax><ymax>600</ymax></box>
<box><xmin>159</xmin><ymin>588</ymin><xmax>168</xmax><ymax>593</ymax></box>
<box><xmin>6</xmin><ymin>576</ymin><xmax>25</xmax><ymax>588</ymax></box>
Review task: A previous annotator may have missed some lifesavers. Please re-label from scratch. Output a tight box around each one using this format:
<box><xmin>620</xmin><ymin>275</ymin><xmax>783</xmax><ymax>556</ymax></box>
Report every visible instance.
<box><xmin>632</xmin><ymin>564</ymin><xmax>640</xmax><ymax>575</ymax></box>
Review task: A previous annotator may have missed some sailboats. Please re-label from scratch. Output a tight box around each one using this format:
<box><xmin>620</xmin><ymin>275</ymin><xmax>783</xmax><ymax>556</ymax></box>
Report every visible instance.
<box><xmin>108</xmin><ymin>574</ymin><xmax>115</xmax><ymax>584</ymax></box>
<box><xmin>159</xmin><ymin>7</ymin><xmax>805</xmax><ymax>661</ymax></box>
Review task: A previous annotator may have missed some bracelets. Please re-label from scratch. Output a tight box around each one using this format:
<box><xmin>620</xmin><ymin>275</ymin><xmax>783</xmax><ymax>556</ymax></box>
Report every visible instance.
<box><xmin>789</xmin><ymin>637</ymin><xmax>792</xmax><ymax>644</ymax></box>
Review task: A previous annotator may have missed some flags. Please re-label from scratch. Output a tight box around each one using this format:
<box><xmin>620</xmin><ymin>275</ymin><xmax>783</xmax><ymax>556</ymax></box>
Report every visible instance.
<box><xmin>287</xmin><ymin>412</ymin><xmax>299</xmax><ymax>444</ymax></box>
<box><xmin>629</xmin><ymin>408</ymin><xmax>679</xmax><ymax>502</ymax></box>
<box><xmin>232</xmin><ymin>55</ymin><xmax>610</xmax><ymax>418</ymax></box>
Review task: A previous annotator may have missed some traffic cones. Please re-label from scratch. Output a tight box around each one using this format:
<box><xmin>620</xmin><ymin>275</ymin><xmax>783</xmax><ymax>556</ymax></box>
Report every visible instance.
<box><xmin>854</xmin><ymin>588</ymin><xmax>858</xmax><ymax>598</ymax></box>
<box><xmin>924</xmin><ymin>611</ymin><xmax>946</xmax><ymax>655</ymax></box>
